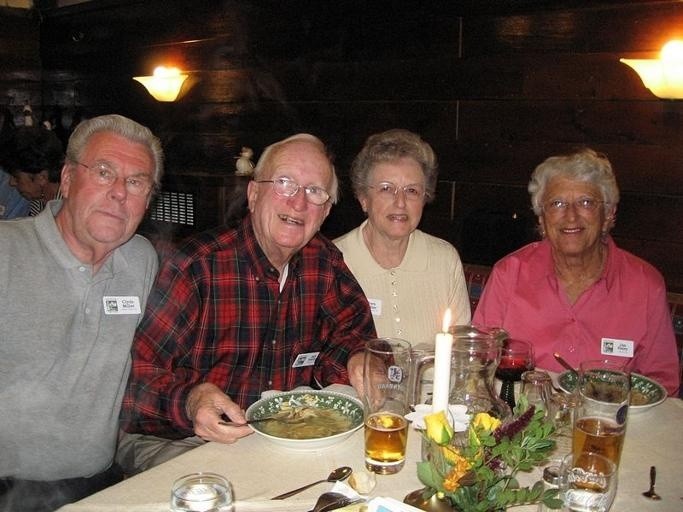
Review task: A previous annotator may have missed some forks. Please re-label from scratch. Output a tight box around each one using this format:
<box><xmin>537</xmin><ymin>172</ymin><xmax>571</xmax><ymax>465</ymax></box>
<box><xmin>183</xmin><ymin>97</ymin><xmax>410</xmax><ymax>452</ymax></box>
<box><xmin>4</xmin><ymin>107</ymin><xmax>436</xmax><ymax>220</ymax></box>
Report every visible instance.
<box><xmin>311</xmin><ymin>491</ymin><xmax>349</xmax><ymax>512</ymax></box>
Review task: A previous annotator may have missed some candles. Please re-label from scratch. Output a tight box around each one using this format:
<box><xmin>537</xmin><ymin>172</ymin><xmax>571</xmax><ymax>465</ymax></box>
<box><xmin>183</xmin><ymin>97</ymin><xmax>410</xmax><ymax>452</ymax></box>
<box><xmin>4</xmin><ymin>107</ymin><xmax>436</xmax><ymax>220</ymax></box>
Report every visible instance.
<box><xmin>432</xmin><ymin>307</ymin><xmax>454</xmax><ymax>415</ymax></box>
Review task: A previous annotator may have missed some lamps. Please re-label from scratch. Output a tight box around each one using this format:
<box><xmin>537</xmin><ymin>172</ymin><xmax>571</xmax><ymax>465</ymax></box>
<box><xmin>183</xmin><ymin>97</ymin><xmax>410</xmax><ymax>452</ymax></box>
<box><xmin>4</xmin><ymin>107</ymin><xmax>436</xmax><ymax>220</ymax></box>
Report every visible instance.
<box><xmin>131</xmin><ymin>62</ymin><xmax>189</xmax><ymax>102</ymax></box>
<box><xmin>618</xmin><ymin>37</ymin><xmax>682</xmax><ymax>131</ymax></box>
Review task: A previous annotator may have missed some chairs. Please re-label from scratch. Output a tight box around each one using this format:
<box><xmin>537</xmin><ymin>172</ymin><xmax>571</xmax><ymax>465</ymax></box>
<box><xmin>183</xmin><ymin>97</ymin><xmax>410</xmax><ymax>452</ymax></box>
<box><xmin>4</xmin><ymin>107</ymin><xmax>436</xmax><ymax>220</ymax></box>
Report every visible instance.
<box><xmin>460</xmin><ymin>259</ymin><xmax>492</xmax><ymax>320</ymax></box>
<box><xmin>665</xmin><ymin>289</ymin><xmax>681</xmax><ymax>390</ymax></box>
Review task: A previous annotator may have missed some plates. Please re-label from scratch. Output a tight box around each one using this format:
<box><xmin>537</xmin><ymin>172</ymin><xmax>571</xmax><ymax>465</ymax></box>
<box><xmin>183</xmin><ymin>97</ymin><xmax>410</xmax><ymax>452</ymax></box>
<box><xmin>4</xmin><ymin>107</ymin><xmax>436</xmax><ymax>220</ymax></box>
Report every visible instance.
<box><xmin>556</xmin><ymin>366</ymin><xmax>668</xmax><ymax>414</ymax></box>
<box><xmin>246</xmin><ymin>390</ymin><xmax>364</xmax><ymax>446</ymax></box>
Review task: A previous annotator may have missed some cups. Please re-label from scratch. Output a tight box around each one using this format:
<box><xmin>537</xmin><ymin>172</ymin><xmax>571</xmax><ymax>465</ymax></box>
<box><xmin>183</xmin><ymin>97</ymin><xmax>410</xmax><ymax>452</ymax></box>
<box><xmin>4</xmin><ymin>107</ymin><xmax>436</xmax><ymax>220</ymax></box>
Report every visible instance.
<box><xmin>516</xmin><ymin>369</ymin><xmax>554</xmax><ymax>431</ymax></box>
<box><xmin>494</xmin><ymin>338</ymin><xmax>534</xmax><ymax>416</ymax></box>
<box><xmin>169</xmin><ymin>472</ymin><xmax>235</xmax><ymax>512</ymax></box>
<box><xmin>363</xmin><ymin>337</ymin><xmax>412</xmax><ymax>473</ymax></box>
<box><xmin>559</xmin><ymin>450</ymin><xmax>618</xmax><ymax>511</ymax></box>
<box><xmin>572</xmin><ymin>360</ymin><xmax>631</xmax><ymax>490</ymax></box>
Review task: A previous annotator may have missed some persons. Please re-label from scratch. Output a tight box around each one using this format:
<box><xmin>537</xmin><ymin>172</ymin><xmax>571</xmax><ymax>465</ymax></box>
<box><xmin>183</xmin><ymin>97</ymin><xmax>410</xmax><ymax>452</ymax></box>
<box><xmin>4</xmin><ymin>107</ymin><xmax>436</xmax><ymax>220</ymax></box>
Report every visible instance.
<box><xmin>330</xmin><ymin>129</ymin><xmax>471</xmax><ymax>396</ymax></box>
<box><xmin>472</xmin><ymin>151</ymin><xmax>681</xmax><ymax>405</ymax></box>
<box><xmin>0</xmin><ymin>117</ymin><xmax>158</xmax><ymax>506</ymax></box>
<box><xmin>0</xmin><ymin>166</ymin><xmax>29</xmax><ymax>220</ymax></box>
<box><xmin>116</xmin><ymin>137</ymin><xmax>388</xmax><ymax>474</ymax></box>
<box><xmin>6</xmin><ymin>129</ymin><xmax>65</xmax><ymax>214</ymax></box>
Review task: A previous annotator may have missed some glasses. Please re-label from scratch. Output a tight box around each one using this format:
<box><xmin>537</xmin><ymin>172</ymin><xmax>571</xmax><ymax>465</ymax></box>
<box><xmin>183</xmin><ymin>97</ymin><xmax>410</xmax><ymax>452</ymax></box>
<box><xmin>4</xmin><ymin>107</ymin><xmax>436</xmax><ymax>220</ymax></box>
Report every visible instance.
<box><xmin>71</xmin><ymin>158</ymin><xmax>153</xmax><ymax>196</ymax></box>
<box><xmin>363</xmin><ymin>180</ymin><xmax>425</xmax><ymax>200</ymax></box>
<box><xmin>255</xmin><ymin>176</ymin><xmax>333</xmax><ymax>206</ymax></box>
<box><xmin>541</xmin><ymin>197</ymin><xmax>606</xmax><ymax>214</ymax></box>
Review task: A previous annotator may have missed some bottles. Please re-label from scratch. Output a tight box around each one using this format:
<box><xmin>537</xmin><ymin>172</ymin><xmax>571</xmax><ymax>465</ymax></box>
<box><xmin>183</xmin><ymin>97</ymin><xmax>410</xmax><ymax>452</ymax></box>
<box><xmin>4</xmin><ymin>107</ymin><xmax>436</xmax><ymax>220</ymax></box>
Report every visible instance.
<box><xmin>537</xmin><ymin>466</ymin><xmax>565</xmax><ymax>512</ymax></box>
<box><xmin>23</xmin><ymin>100</ymin><xmax>31</xmax><ymax>126</ymax></box>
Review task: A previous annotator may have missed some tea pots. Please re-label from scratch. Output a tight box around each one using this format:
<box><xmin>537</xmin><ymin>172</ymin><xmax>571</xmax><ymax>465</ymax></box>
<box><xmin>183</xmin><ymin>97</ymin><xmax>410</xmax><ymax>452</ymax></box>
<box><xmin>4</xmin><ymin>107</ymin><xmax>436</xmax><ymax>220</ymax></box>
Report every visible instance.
<box><xmin>411</xmin><ymin>324</ymin><xmax>508</xmax><ymax>460</ymax></box>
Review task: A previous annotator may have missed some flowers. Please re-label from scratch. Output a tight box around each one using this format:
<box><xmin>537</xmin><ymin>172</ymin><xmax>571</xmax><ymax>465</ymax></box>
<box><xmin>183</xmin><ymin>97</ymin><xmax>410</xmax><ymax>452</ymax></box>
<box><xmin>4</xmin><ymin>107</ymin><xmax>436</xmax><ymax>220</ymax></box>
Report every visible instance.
<box><xmin>415</xmin><ymin>402</ymin><xmax>564</xmax><ymax>512</ymax></box>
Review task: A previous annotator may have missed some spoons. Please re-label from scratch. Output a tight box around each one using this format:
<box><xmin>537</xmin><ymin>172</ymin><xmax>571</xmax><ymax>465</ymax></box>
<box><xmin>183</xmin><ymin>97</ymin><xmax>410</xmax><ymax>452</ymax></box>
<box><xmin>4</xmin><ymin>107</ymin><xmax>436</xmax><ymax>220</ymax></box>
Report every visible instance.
<box><xmin>553</xmin><ymin>353</ymin><xmax>613</xmax><ymax>403</ymax></box>
<box><xmin>642</xmin><ymin>464</ymin><xmax>662</xmax><ymax>501</ymax></box>
<box><xmin>271</xmin><ymin>467</ymin><xmax>352</xmax><ymax>501</ymax></box>
<box><xmin>217</xmin><ymin>415</ymin><xmax>303</xmax><ymax>428</ymax></box>
